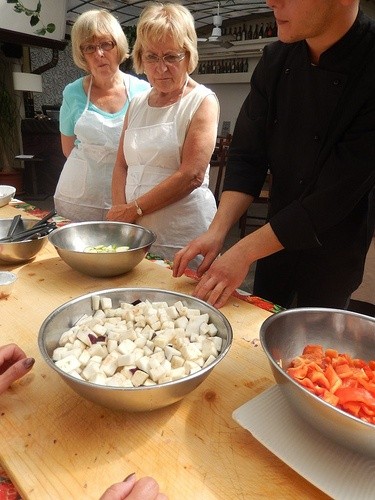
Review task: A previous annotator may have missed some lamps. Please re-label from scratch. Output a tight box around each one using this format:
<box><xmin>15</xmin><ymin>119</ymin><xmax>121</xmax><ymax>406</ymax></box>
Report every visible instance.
<box><xmin>12</xmin><ymin>72</ymin><xmax>42</xmax><ymax>119</ymax></box>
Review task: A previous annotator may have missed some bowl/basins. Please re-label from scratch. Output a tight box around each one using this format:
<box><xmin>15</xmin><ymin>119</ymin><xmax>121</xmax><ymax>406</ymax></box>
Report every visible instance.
<box><xmin>0</xmin><ymin>185</ymin><xmax>16</xmax><ymax>206</ymax></box>
<box><xmin>47</xmin><ymin>221</ymin><xmax>157</xmax><ymax>278</ymax></box>
<box><xmin>37</xmin><ymin>287</ymin><xmax>234</xmax><ymax>411</ymax></box>
<box><xmin>259</xmin><ymin>307</ymin><xmax>375</xmax><ymax>460</ymax></box>
<box><xmin>0</xmin><ymin>216</ymin><xmax>59</xmax><ymax>264</ymax></box>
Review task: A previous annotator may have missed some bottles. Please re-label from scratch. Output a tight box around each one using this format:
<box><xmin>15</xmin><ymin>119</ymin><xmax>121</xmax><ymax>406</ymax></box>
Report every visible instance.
<box><xmin>198</xmin><ymin>58</ymin><xmax>248</xmax><ymax>74</ymax></box>
<box><xmin>221</xmin><ymin>21</ymin><xmax>278</xmax><ymax>41</ymax></box>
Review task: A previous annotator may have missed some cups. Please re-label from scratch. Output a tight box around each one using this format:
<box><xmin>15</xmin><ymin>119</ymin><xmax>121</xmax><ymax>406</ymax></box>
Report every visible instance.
<box><xmin>0</xmin><ymin>271</ymin><xmax>16</xmax><ymax>297</ymax></box>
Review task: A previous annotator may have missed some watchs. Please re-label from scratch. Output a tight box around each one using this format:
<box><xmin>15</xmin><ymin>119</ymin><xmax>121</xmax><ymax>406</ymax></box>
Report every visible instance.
<box><xmin>134</xmin><ymin>199</ymin><xmax>143</xmax><ymax>217</ymax></box>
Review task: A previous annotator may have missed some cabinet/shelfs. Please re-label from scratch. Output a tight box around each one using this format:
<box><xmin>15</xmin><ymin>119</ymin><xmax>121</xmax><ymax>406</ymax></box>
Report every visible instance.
<box><xmin>188</xmin><ymin>37</ymin><xmax>279</xmax><ymax>84</ymax></box>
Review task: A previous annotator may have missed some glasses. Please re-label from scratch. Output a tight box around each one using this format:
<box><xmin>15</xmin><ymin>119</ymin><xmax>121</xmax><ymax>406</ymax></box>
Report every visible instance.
<box><xmin>141</xmin><ymin>51</ymin><xmax>189</xmax><ymax>63</ymax></box>
<box><xmin>81</xmin><ymin>40</ymin><xmax>116</xmax><ymax>55</ymax></box>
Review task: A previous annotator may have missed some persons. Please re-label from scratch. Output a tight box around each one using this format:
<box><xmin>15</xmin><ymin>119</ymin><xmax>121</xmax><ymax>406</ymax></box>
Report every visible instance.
<box><xmin>173</xmin><ymin>0</ymin><xmax>375</xmax><ymax>316</ymax></box>
<box><xmin>53</xmin><ymin>9</ymin><xmax>153</xmax><ymax>223</ymax></box>
<box><xmin>107</xmin><ymin>0</ymin><xmax>221</xmax><ymax>274</ymax></box>
<box><xmin>0</xmin><ymin>343</ymin><xmax>168</xmax><ymax>500</ymax></box>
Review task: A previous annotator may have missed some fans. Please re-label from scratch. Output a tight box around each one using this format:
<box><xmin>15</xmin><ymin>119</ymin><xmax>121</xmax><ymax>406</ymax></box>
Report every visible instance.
<box><xmin>198</xmin><ymin>4</ymin><xmax>236</xmax><ymax>49</ymax></box>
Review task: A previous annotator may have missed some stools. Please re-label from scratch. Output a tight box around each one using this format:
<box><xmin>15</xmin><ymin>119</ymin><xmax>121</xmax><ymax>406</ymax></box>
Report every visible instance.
<box><xmin>16</xmin><ymin>154</ymin><xmax>50</xmax><ymax>200</ymax></box>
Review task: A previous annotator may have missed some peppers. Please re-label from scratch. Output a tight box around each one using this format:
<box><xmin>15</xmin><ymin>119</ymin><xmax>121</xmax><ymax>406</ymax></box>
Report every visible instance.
<box><xmin>285</xmin><ymin>343</ymin><xmax>375</xmax><ymax>430</ymax></box>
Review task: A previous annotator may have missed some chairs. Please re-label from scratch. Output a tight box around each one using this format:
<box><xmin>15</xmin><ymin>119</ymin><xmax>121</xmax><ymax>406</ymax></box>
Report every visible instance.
<box><xmin>209</xmin><ymin>133</ymin><xmax>272</xmax><ymax>239</ymax></box>
